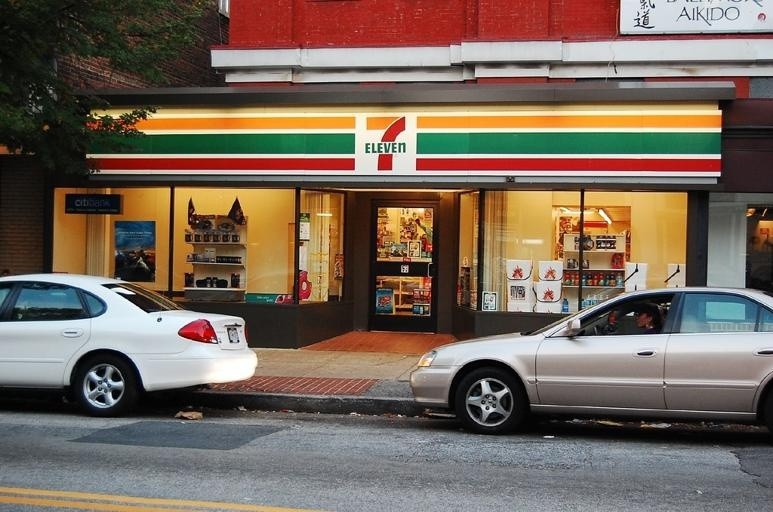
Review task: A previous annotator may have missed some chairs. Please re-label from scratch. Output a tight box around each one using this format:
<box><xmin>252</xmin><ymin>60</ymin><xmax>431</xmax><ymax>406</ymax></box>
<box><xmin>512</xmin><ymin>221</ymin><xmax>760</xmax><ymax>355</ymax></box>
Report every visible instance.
<box><xmin>659</xmin><ymin>301</ymin><xmax>710</xmax><ymax>335</ymax></box>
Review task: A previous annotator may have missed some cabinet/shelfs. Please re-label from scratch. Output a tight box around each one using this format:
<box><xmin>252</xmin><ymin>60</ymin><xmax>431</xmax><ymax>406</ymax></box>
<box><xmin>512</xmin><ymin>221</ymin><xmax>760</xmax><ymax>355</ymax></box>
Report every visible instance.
<box><xmin>376</xmin><ymin>275</ymin><xmax>432</xmax><ymax>312</ymax></box>
<box><xmin>558</xmin><ymin>234</ymin><xmax>628</xmax><ymax>306</ymax></box>
<box><xmin>181</xmin><ymin>214</ymin><xmax>247</xmax><ymax>301</ymax></box>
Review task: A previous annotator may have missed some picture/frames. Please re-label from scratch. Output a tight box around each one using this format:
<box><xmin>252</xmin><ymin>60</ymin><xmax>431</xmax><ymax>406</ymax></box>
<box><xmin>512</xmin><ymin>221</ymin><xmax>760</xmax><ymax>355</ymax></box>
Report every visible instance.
<box><xmin>406</xmin><ymin>239</ymin><xmax>423</xmax><ymax>259</ymax></box>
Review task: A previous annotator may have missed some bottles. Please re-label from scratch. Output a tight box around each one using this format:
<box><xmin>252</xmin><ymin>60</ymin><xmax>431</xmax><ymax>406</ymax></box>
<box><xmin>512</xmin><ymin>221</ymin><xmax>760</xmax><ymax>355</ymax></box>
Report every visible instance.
<box><xmin>581</xmin><ymin>293</ymin><xmax>609</xmax><ymax>310</ymax></box>
<box><xmin>596</xmin><ymin>235</ymin><xmax>616</xmax><ymax>248</ymax></box>
<box><xmin>206</xmin><ymin>277</ymin><xmax>217</xmax><ymax>288</ymax></box>
<box><xmin>562</xmin><ymin>298</ymin><xmax>570</xmax><ymax>312</ymax></box>
<box><xmin>564</xmin><ymin>270</ymin><xmax>624</xmax><ymax>286</ymax></box>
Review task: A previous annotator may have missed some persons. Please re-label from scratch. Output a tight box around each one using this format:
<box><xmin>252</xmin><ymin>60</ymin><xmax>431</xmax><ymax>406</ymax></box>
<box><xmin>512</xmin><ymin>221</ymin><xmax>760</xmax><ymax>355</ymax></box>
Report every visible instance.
<box><xmin>636</xmin><ymin>303</ymin><xmax>662</xmax><ymax>334</ymax></box>
<box><xmin>415</xmin><ymin>218</ymin><xmax>433</xmax><ymax>244</ymax></box>
<box><xmin>116</xmin><ymin>249</ymin><xmax>155</xmax><ymax>281</ymax></box>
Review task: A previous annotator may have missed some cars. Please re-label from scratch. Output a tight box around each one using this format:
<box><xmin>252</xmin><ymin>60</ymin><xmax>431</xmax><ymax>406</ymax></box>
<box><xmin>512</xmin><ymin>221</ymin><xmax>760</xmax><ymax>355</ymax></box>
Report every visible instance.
<box><xmin>470</xmin><ymin>237</ymin><xmax>543</xmax><ymax>307</ymax></box>
<box><xmin>410</xmin><ymin>286</ymin><xmax>773</xmax><ymax>434</ymax></box>
<box><xmin>0</xmin><ymin>272</ymin><xmax>258</xmax><ymax>417</ymax></box>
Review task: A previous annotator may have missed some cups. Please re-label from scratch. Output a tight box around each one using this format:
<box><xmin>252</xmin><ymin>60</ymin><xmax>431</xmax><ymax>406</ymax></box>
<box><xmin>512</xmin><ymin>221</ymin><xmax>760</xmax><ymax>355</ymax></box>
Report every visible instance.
<box><xmin>185</xmin><ymin>232</ymin><xmax>240</xmax><ymax>242</ymax></box>
